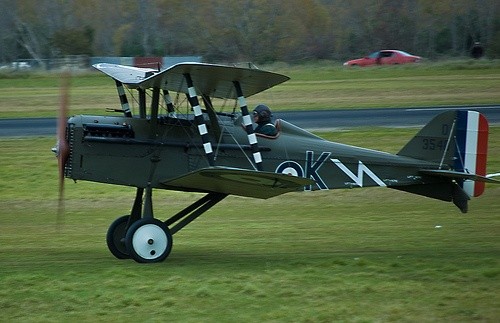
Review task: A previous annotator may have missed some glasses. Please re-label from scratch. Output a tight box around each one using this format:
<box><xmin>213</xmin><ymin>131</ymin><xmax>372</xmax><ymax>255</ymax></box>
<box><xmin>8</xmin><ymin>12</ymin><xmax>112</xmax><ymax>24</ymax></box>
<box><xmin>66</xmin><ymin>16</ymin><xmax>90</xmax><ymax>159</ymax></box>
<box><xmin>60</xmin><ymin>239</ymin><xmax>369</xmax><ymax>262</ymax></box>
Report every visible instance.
<box><xmin>253</xmin><ymin>111</ymin><xmax>258</xmax><ymax>117</ymax></box>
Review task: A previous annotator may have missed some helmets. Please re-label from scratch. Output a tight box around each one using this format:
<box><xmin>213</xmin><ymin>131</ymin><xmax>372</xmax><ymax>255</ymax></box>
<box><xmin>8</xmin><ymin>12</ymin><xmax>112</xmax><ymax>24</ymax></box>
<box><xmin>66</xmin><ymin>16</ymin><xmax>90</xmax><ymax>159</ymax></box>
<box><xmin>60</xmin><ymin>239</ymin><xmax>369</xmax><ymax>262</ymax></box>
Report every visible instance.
<box><xmin>253</xmin><ymin>104</ymin><xmax>272</xmax><ymax>124</ymax></box>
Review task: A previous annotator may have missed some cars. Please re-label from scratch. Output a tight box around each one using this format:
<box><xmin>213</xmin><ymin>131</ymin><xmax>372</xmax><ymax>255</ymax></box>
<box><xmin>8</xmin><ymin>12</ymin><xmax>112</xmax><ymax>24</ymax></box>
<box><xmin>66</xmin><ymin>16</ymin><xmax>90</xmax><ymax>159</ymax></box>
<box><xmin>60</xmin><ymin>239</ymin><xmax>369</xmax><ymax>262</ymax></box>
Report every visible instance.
<box><xmin>343</xmin><ymin>49</ymin><xmax>423</xmax><ymax>65</ymax></box>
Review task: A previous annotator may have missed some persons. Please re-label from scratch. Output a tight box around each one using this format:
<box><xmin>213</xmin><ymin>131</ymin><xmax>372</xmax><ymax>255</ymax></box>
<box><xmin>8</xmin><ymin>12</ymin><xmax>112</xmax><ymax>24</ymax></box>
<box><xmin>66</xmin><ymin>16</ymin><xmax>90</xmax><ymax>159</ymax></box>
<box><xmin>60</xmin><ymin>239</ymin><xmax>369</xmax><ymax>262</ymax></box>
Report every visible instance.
<box><xmin>254</xmin><ymin>103</ymin><xmax>279</xmax><ymax>138</ymax></box>
<box><xmin>471</xmin><ymin>41</ymin><xmax>484</xmax><ymax>58</ymax></box>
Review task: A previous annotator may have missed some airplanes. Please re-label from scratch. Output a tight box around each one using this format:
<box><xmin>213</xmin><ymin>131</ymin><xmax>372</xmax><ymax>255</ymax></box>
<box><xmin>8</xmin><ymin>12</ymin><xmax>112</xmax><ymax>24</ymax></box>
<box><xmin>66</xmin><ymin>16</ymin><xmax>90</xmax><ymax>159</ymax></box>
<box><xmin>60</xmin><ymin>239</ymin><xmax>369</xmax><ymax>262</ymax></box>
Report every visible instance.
<box><xmin>50</xmin><ymin>61</ymin><xmax>496</xmax><ymax>264</ymax></box>
